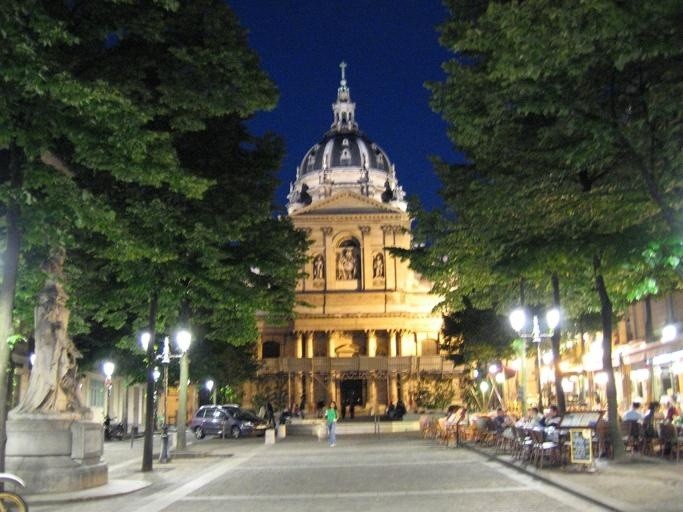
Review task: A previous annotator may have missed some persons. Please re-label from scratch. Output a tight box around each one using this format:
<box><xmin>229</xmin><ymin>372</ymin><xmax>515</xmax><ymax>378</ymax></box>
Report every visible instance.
<box><xmin>317</xmin><ymin>400</ymin><xmax>323</xmax><ymax>418</ymax></box>
<box><xmin>493</xmin><ymin>400</ymin><xmax>683</xmax><ymax>439</ymax></box>
<box><xmin>324</xmin><ymin>401</ymin><xmax>339</xmax><ymax>447</ymax></box>
<box><xmin>373</xmin><ymin>255</ymin><xmax>383</xmax><ymax>278</ymax></box>
<box><xmin>338</xmin><ymin>250</ymin><xmax>357</xmax><ymax>279</ymax></box>
<box><xmin>448</xmin><ymin>408</ymin><xmax>463</xmax><ymax>424</ymax></box>
<box><xmin>280</xmin><ymin>409</ymin><xmax>290</xmax><ymax>424</ymax></box>
<box><xmin>387</xmin><ymin>398</ymin><xmax>406</xmax><ymax>420</ymax></box>
<box><xmin>340</xmin><ymin>398</ymin><xmax>355</xmax><ymax>419</ymax></box>
<box><xmin>574</xmin><ymin>432</ymin><xmax>587</xmax><ymax>460</ymax></box>
<box><xmin>267</xmin><ymin>400</ymin><xmax>276</xmax><ymax>429</ymax></box>
<box><xmin>313</xmin><ymin>255</ymin><xmax>324</xmax><ymax>278</ymax></box>
<box><xmin>294</xmin><ymin>397</ymin><xmax>304</xmax><ymax>420</ymax></box>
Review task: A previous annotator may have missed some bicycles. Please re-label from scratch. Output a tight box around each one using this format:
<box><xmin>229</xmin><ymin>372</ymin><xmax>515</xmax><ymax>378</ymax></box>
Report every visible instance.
<box><xmin>0</xmin><ymin>473</ymin><xmax>30</xmax><ymax>512</ymax></box>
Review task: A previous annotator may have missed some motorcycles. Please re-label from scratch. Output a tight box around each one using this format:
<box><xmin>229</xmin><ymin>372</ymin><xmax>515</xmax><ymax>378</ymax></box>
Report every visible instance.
<box><xmin>103</xmin><ymin>415</ymin><xmax>126</xmax><ymax>442</ymax></box>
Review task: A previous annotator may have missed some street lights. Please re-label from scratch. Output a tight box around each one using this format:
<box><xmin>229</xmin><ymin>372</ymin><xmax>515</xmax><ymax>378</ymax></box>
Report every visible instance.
<box><xmin>102</xmin><ymin>361</ymin><xmax>115</xmax><ymax>427</ymax></box>
<box><xmin>509</xmin><ymin>307</ymin><xmax>561</xmax><ymax>412</ymax></box>
<box><xmin>142</xmin><ymin>329</ymin><xmax>192</xmax><ymax>465</ymax></box>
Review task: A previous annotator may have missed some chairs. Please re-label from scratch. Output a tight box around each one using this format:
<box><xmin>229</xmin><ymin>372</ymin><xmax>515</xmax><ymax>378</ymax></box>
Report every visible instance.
<box><xmin>472</xmin><ymin>412</ymin><xmax>683</xmax><ymax>472</ymax></box>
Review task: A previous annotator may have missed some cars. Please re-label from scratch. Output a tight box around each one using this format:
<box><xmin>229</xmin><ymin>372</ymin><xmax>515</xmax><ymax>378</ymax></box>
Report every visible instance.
<box><xmin>189</xmin><ymin>404</ymin><xmax>267</xmax><ymax>440</ymax></box>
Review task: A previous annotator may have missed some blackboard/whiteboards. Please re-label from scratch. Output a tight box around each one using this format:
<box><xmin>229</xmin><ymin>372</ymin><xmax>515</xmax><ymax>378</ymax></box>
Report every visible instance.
<box><xmin>569</xmin><ymin>428</ymin><xmax>592</xmax><ymax>464</ymax></box>
